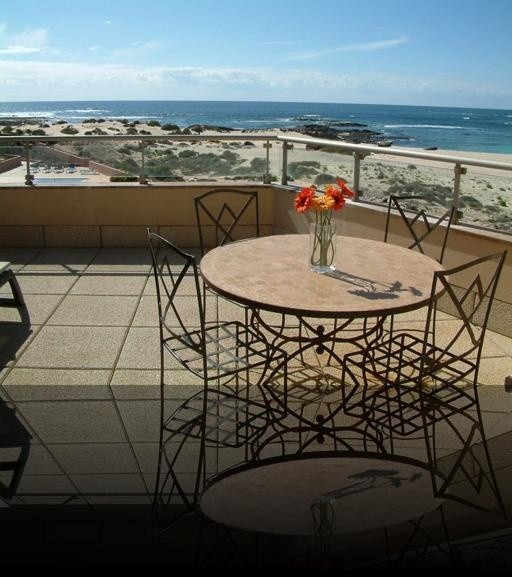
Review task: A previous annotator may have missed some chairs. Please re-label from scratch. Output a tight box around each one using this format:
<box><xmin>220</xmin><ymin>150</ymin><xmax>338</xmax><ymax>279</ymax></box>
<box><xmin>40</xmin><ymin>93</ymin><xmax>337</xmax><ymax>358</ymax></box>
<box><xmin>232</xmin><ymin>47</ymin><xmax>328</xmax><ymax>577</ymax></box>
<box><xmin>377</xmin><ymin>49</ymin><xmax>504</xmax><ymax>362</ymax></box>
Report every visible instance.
<box><xmin>338</xmin><ymin>383</ymin><xmax>506</xmax><ymax>526</ymax></box>
<box><xmin>192</xmin><ymin>185</ymin><xmax>311</xmax><ymax>367</ymax></box>
<box><xmin>150</xmin><ymin>388</ymin><xmax>278</xmax><ymax>533</ymax></box>
<box><xmin>142</xmin><ymin>223</ymin><xmax>289</xmax><ymax>411</ymax></box>
<box><xmin>376</xmin><ymin>193</ymin><xmax>460</xmax><ymax>362</ymax></box>
<box><xmin>340</xmin><ymin>247</ymin><xmax>510</xmax><ymax>449</ymax></box>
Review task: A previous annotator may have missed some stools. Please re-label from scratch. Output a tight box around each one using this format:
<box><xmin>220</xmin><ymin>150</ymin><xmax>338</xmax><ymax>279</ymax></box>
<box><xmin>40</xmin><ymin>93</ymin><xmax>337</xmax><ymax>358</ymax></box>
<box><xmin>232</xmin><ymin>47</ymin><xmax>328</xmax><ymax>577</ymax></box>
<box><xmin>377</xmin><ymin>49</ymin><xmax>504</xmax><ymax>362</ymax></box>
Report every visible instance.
<box><xmin>1</xmin><ymin>256</ymin><xmax>32</xmax><ymax>374</ymax></box>
<box><xmin>0</xmin><ymin>398</ymin><xmax>37</xmax><ymax>503</ymax></box>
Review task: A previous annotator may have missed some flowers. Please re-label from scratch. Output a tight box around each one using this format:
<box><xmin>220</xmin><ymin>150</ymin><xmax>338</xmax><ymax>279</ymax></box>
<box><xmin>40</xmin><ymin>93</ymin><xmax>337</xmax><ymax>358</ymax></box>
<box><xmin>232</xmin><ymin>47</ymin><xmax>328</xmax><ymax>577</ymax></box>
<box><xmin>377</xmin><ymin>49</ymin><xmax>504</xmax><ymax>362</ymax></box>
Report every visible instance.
<box><xmin>290</xmin><ymin>176</ymin><xmax>353</xmax><ymax>262</ymax></box>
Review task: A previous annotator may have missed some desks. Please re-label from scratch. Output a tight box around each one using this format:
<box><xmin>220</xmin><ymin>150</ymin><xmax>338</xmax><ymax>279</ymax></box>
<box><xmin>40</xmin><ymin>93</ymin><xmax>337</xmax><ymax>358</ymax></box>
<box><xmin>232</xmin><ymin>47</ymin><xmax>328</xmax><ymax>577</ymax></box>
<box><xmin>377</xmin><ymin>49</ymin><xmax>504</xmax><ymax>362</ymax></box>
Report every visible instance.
<box><xmin>196</xmin><ymin>231</ymin><xmax>453</xmax><ymax>389</ymax></box>
<box><xmin>198</xmin><ymin>414</ymin><xmax>454</xmax><ymax>537</ymax></box>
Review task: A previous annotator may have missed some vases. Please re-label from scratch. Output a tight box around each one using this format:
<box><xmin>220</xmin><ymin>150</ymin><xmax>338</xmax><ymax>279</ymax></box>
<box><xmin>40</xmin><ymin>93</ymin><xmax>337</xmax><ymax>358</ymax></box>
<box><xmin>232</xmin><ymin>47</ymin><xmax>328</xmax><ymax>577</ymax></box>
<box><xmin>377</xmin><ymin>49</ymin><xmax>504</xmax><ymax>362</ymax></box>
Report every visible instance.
<box><xmin>306</xmin><ymin>216</ymin><xmax>341</xmax><ymax>276</ymax></box>
